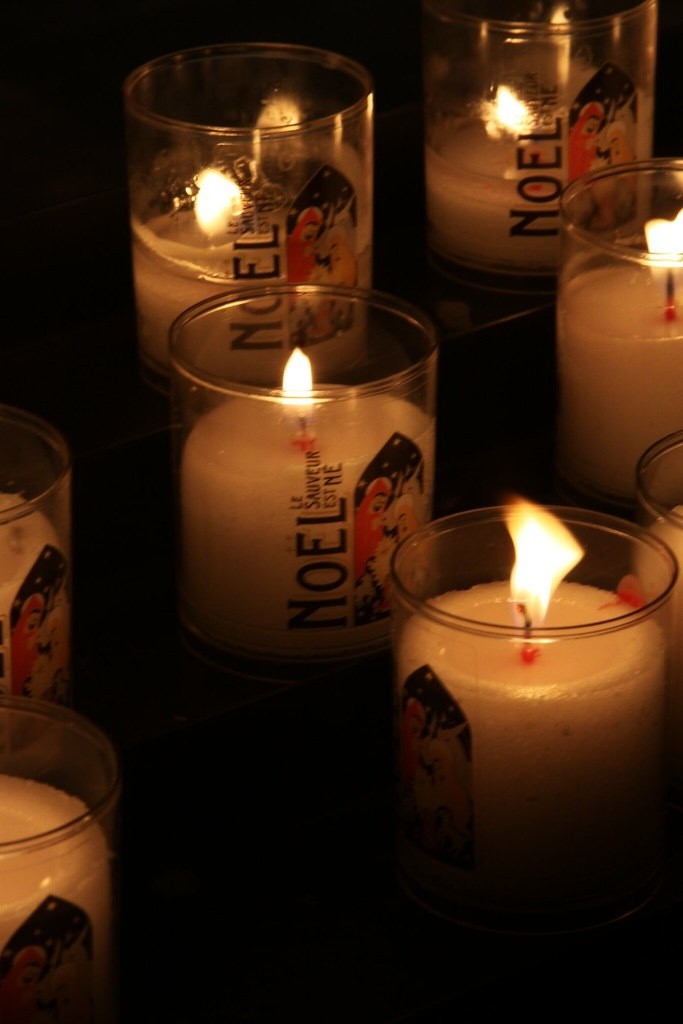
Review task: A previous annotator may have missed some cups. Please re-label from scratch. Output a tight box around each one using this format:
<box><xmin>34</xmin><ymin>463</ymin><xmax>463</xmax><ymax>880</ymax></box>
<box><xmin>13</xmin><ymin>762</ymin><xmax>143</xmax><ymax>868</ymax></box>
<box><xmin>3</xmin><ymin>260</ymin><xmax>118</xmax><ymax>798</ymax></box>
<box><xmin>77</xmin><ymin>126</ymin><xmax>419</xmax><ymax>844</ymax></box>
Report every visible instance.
<box><xmin>635</xmin><ymin>429</ymin><xmax>683</xmax><ymax>811</ymax></box>
<box><xmin>0</xmin><ymin>402</ymin><xmax>72</xmax><ymax>709</ymax></box>
<box><xmin>127</xmin><ymin>44</ymin><xmax>373</xmax><ymax>394</ymax></box>
<box><xmin>424</xmin><ymin>1</ymin><xmax>657</xmax><ymax>294</ymax></box>
<box><xmin>390</xmin><ymin>503</ymin><xmax>678</xmax><ymax>936</ymax></box>
<box><xmin>0</xmin><ymin>699</ymin><xmax>122</xmax><ymax>1024</ymax></box>
<box><xmin>560</xmin><ymin>157</ymin><xmax>683</xmax><ymax>517</ymax></box>
<box><xmin>169</xmin><ymin>283</ymin><xmax>436</xmax><ymax>685</ymax></box>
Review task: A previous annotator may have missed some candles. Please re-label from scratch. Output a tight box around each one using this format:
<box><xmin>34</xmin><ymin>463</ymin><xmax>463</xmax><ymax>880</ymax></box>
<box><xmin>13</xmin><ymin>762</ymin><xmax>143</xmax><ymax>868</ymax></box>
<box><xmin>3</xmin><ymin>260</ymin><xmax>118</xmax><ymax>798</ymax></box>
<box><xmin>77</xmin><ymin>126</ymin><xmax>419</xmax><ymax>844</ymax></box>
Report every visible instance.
<box><xmin>402</xmin><ymin>496</ymin><xmax>664</xmax><ymax>852</ymax></box>
<box><xmin>429</xmin><ymin>83</ymin><xmax>650</xmax><ymax>268</ymax></box>
<box><xmin>131</xmin><ymin>102</ymin><xmax>370</xmax><ymax>374</ymax></box>
<box><xmin>558</xmin><ymin>204</ymin><xmax>683</xmax><ymax>499</ymax></box>
<box><xmin>633</xmin><ymin>504</ymin><xmax>683</xmax><ymax>699</ymax></box>
<box><xmin>0</xmin><ymin>493</ymin><xmax>68</xmax><ymax>696</ymax></box>
<box><xmin>181</xmin><ymin>347</ymin><xmax>433</xmax><ymax>629</ymax></box>
<box><xmin>0</xmin><ymin>773</ymin><xmax>109</xmax><ymax>965</ymax></box>
